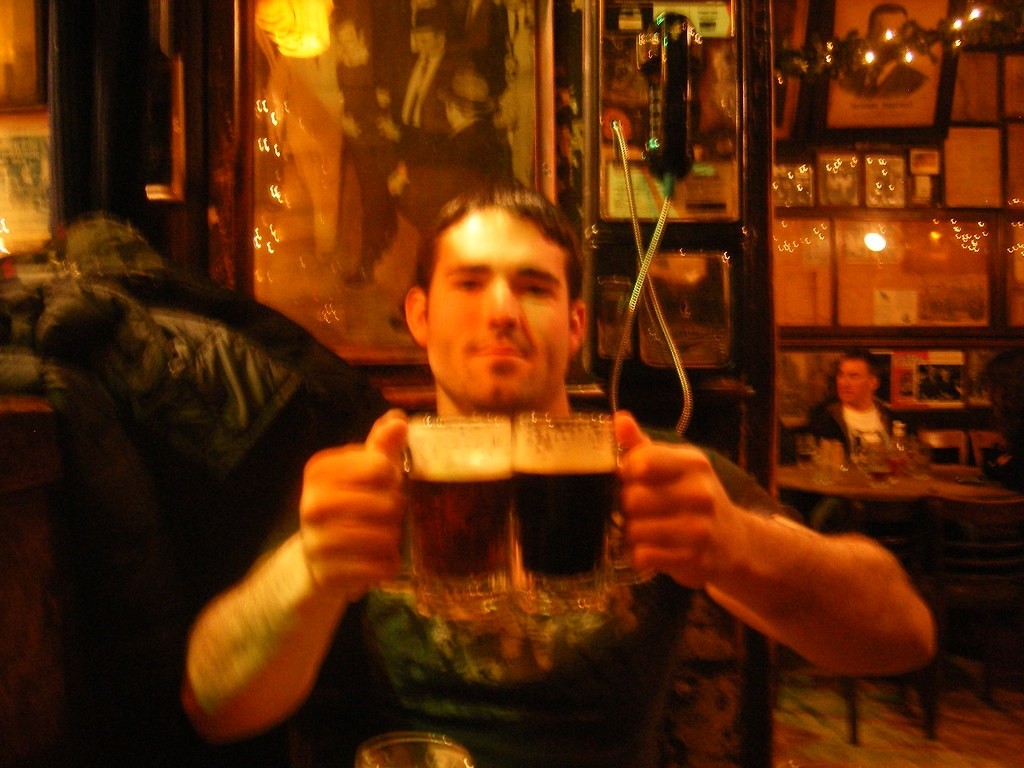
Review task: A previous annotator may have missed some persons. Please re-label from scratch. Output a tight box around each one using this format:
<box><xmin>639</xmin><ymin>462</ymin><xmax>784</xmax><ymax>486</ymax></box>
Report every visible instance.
<box><xmin>920</xmin><ymin>367</ymin><xmax>949</xmax><ymax>399</ymax></box>
<box><xmin>940</xmin><ymin>368</ymin><xmax>961</xmax><ymax>399</ymax></box>
<box><xmin>958</xmin><ymin>348</ymin><xmax>1024</xmax><ymax>495</ymax></box>
<box><xmin>0</xmin><ymin>208</ymin><xmax>379</xmax><ymax>767</ymax></box>
<box><xmin>332</xmin><ymin>0</ymin><xmax>515</xmax><ymax>337</ymax></box>
<box><xmin>178</xmin><ymin>185</ymin><xmax>935</xmax><ymax>767</ymax></box>
<box><xmin>799</xmin><ymin>350</ymin><xmax>918</xmax><ymax>465</ymax></box>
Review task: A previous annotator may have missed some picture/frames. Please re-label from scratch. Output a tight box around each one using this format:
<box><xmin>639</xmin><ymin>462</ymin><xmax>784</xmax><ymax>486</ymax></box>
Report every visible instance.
<box><xmin>368</xmin><ymin>0</ymin><xmax>604</xmax><ymax>404</ymax></box>
<box><xmin>631</xmin><ymin>240</ymin><xmax>738</xmax><ymax>376</ymax></box>
<box><xmin>0</xmin><ymin>104</ymin><xmax>58</xmax><ymax>263</ymax></box>
<box><xmin>246</xmin><ymin>0</ymin><xmax>557</xmax><ymax>369</ymax></box>
<box><xmin>772</xmin><ymin>0</ymin><xmax>819</xmax><ymax>153</ymax></box>
<box><xmin>809</xmin><ymin>1</ymin><xmax>967</xmax><ymax>142</ymax></box>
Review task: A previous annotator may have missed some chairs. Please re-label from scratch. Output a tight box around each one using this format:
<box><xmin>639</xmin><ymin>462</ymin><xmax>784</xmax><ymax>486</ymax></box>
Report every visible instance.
<box><xmin>831</xmin><ymin>493</ymin><xmax>937</xmax><ymax>745</ymax></box>
<box><xmin>915</xmin><ymin>429</ymin><xmax>966</xmax><ymax>466</ymax></box>
<box><xmin>299</xmin><ymin>592</ymin><xmax>782</xmax><ymax>768</ymax></box>
<box><xmin>966</xmin><ymin>430</ymin><xmax>1015</xmax><ymax>465</ymax></box>
<box><xmin>921</xmin><ymin>489</ymin><xmax>1024</xmax><ymax>740</ymax></box>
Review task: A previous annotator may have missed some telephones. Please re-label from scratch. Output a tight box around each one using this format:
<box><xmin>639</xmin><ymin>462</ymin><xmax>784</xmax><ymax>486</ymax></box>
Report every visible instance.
<box><xmin>598</xmin><ymin>1</ymin><xmax>744</xmax><ymax>224</ymax></box>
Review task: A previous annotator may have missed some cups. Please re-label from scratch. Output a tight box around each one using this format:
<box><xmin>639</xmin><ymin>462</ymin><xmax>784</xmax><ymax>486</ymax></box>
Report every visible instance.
<box><xmin>375</xmin><ymin>413</ymin><xmax>513</xmax><ymax>618</ymax></box>
<box><xmin>513</xmin><ymin>413</ymin><xmax>661</xmax><ymax>615</ymax></box>
<box><xmin>796</xmin><ymin>432</ymin><xmax>934</xmax><ymax>489</ymax></box>
<box><xmin>356</xmin><ymin>730</ymin><xmax>472</xmax><ymax>768</ymax></box>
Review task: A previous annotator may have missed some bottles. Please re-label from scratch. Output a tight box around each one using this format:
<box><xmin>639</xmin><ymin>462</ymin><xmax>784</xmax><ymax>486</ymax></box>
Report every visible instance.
<box><xmin>888</xmin><ymin>421</ymin><xmax>909</xmax><ymax>484</ymax></box>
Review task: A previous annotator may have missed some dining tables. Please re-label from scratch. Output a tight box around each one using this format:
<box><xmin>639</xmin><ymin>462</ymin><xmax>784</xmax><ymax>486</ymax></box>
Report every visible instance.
<box><xmin>777</xmin><ymin>464</ymin><xmax>1023</xmax><ymax>744</ymax></box>
